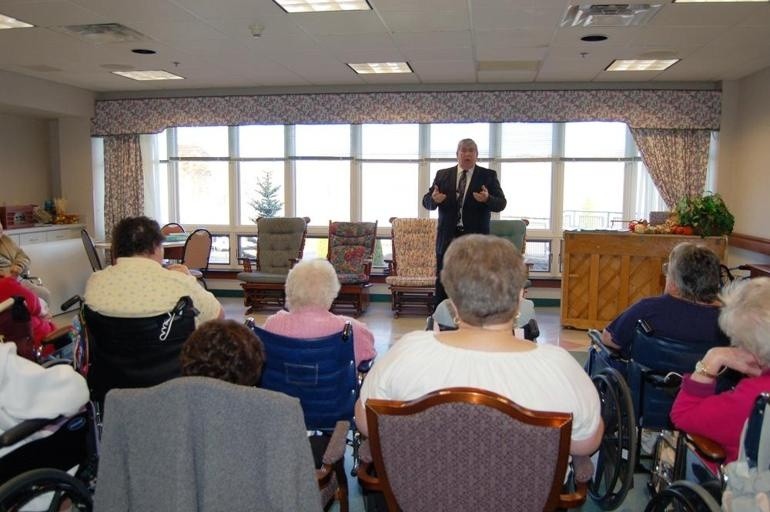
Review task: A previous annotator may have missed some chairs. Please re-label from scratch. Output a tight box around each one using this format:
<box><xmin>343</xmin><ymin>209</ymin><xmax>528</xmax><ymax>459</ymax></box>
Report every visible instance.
<box><xmin>489</xmin><ymin>220</ymin><xmax>526</xmax><ymax>256</ymax></box>
<box><xmin>161</xmin><ymin>223</ymin><xmax>184</xmax><ymax>236</ymax></box>
<box><xmin>102</xmin><ymin>376</ymin><xmax>350</xmax><ymax>512</ymax></box>
<box><xmin>236</xmin><ymin>215</ymin><xmax>311</xmax><ymax>315</ymax></box>
<box><xmin>325</xmin><ymin>219</ymin><xmax>378</xmax><ymax>318</ymax></box>
<box><xmin>384</xmin><ymin>217</ymin><xmax>436</xmax><ymax>317</ymax></box>
<box><xmin>352</xmin><ymin>386</ymin><xmax>596</xmax><ymax>512</ymax></box>
<box><xmin>180</xmin><ymin>228</ymin><xmax>212</xmax><ymax>290</ymax></box>
<box><xmin>80</xmin><ymin>228</ymin><xmax>103</xmax><ymax>272</ymax></box>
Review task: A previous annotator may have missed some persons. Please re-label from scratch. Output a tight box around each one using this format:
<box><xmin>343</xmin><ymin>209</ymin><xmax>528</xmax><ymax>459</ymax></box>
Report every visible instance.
<box><xmin>353</xmin><ymin>234</ymin><xmax>605</xmax><ymax>495</ymax></box>
<box><xmin>261</xmin><ymin>258</ymin><xmax>376</xmax><ymax>377</ymax></box>
<box><xmin>178</xmin><ymin>317</ymin><xmax>265</xmax><ymax>386</ymax></box>
<box><xmin>0</xmin><ymin>340</ymin><xmax>90</xmax><ymax>512</ymax></box>
<box><xmin>432</xmin><ymin>299</ymin><xmax>535</xmax><ymax>328</ymax></box>
<box><xmin>0</xmin><ymin>222</ymin><xmax>51</xmax><ymax>318</ymax></box>
<box><xmin>83</xmin><ymin>215</ymin><xmax>225</xmax><ymax>330</ymax></box>
<box><xmin>421</xmin><ymin>138</ymin><xmax>507</xmax><ymax>315</ymax></box>
<box><xmin>669</xmin><ymin>276</ymin><xmax>770</xmax><ymax>484</ymax></box>
<box><xmin>582</xmin><ymin>242</ymin><xmax>731</xmax><ymax>461</ymax></box>
<box><xmin>0</xmin><ymin>275</ymin><xmax>56</xmax><ymax>359</ymax></box>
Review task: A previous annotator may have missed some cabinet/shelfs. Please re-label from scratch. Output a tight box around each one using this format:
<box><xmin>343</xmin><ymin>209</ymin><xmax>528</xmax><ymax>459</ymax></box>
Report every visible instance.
<box><xmin>2</xmin><ymin>223</ymin><xmax>90</xmax><ymax>317</ymax></box>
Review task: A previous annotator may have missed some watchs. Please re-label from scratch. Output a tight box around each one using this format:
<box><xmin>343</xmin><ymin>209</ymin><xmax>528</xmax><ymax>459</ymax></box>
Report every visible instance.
<box><xmin>695</xmin><ymin>359</ymin><xmax>719</xmax><ymax>379</ymax></box>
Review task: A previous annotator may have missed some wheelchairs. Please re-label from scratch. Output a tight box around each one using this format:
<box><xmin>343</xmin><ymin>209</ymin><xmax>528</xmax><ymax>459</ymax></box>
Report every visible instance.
<box><xmin>641</xmin><ymin>389</ymin><xmax>770</xmax><ymax>511</ymax></box>
<box><xmin>424</xmin><ymin>316</ymin><xmax>542</xmax><ymax>343</ymax></box>
<box><xmin>242</xmin><ymin>315</ymin><xmax>375</xmax><ymax>476</ymax></box>
<box><xmin>2</xmin><ymin>291</ymin><xmax>74</xmax><ymax>374</ymax></box>
<box><xmin>57</xmin><ymin>293</ymin><xmax>199</xmax><ymax>443</ymax></box>
<box><xmin>0</xmin><ymin>398</ymin><xmax>99</xmax><ymax>512</ymax></box>
<box><xmin>579</xmin><ymin>317</ymin><xmax>693</xmax><ymax>512</ymax></box>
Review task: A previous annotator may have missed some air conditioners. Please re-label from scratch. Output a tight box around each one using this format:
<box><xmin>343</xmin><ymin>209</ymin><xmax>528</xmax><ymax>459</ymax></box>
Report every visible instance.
<box><xmin>559</xmin><ymin>2</ymin><xmax>665</xmax><ymax>30</ymax></box>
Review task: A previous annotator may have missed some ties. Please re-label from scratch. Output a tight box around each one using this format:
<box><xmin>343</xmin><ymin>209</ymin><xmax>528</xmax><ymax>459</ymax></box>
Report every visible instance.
<box><xmin>456</xmin><ymin>169</ymin><xmax>468</xmax><ymax>225</ymax></box>
